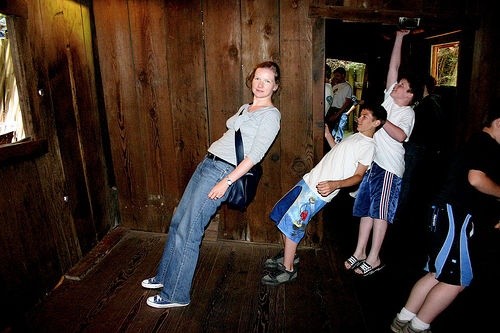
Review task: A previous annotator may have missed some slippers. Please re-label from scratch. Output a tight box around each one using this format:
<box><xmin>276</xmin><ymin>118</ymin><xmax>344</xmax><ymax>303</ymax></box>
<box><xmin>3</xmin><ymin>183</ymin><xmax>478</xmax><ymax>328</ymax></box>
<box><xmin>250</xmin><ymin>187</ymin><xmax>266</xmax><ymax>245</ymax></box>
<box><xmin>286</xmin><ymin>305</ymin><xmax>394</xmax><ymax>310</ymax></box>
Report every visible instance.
<box><xmin>353</xmin><ymin>260</ymin><xmax>385</xmax><ymax>277</ymax></box>
<box><xmin>344</xmin><ymin>255</ymin><xmax>367</xmax><ymax>270</ymax></box>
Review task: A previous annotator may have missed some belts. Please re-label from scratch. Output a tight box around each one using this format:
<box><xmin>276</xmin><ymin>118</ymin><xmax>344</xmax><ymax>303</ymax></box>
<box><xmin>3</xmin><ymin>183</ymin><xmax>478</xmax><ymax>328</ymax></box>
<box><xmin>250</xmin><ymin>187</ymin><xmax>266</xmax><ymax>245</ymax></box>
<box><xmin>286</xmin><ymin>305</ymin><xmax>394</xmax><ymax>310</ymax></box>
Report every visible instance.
<box><xmin>207</xmin><ymin>152</ymin><xmax>236</xmax><ymax>169</ymax></box>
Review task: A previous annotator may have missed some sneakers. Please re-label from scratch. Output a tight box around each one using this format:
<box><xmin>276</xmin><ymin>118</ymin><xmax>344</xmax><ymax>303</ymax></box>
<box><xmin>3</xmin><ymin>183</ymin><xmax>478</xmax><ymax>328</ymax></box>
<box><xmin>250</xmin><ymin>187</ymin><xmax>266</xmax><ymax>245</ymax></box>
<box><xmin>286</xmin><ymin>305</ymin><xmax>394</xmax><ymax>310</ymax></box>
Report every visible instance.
<box><xmin>146</xmin><ymin>294</ymin><xmax>190</xmax><ymax>309</ymax></box>
<box><xmin>264</xmin><ymin>248</ymin><xmax>300</xmax><ymax>269</ymax></box>
<box><xmin>261</xmin><ymin>262</ymin><xmax>298</xmax><ymax>286</ymax></box>
<box><xmin>141</xmin><ymin>277</ymin><xmax>164</xmax><ymax>289</ymax></box>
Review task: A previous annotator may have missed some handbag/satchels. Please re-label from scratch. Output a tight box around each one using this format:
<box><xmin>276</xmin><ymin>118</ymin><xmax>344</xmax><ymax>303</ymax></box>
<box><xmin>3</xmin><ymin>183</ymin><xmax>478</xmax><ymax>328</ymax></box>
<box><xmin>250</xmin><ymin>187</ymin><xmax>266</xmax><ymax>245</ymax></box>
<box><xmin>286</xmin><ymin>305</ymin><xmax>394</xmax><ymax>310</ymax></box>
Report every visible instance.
<box><xmin>227</xmin><ymin>165</ymin><xmax>264</xmax><ymax>212</ymax></box>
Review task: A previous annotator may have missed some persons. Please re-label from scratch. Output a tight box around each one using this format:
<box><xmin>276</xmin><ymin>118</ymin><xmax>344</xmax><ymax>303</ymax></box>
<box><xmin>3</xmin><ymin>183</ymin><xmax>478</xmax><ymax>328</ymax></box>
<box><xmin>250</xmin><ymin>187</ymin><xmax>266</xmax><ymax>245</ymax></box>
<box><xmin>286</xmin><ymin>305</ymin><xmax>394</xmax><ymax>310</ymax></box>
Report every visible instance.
<box><xmin>139</xmin><ymin>61</ymin><xmax>281</xmax><ymax>307</ymax></box>
<box><xmin>391</xmin><ymin>115</ymin><xmax>500</xmax><ymax>333</ymax></box>
<box><xmin>345</xmin><ymin>30</ymin><xmax>418</xmax><ymax>277</ymax></box>
<box><xmin>325</xmin><ymin>64</ymin><xmax>352</xmax><ymax>134</ymax></box>
<box><xmin>260</xmin><ymin>103</ymin><xmax>387</xmax><ymax>286</ymax></box>
<box><xmin>389</xmin><ymin>77</ymin><xmax>442</xmax><ymax>223</ymax></box>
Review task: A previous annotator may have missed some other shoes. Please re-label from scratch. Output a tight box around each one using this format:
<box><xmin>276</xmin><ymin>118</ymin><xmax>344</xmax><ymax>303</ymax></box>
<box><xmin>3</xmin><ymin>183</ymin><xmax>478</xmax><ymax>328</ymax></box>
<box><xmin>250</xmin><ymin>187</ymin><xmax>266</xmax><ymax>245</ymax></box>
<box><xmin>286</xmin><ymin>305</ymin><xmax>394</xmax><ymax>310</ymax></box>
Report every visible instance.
<box><xmin>391</xmin><ymin>317</ymin><xmax>432</xmax><ymax>333</ymax></box>
